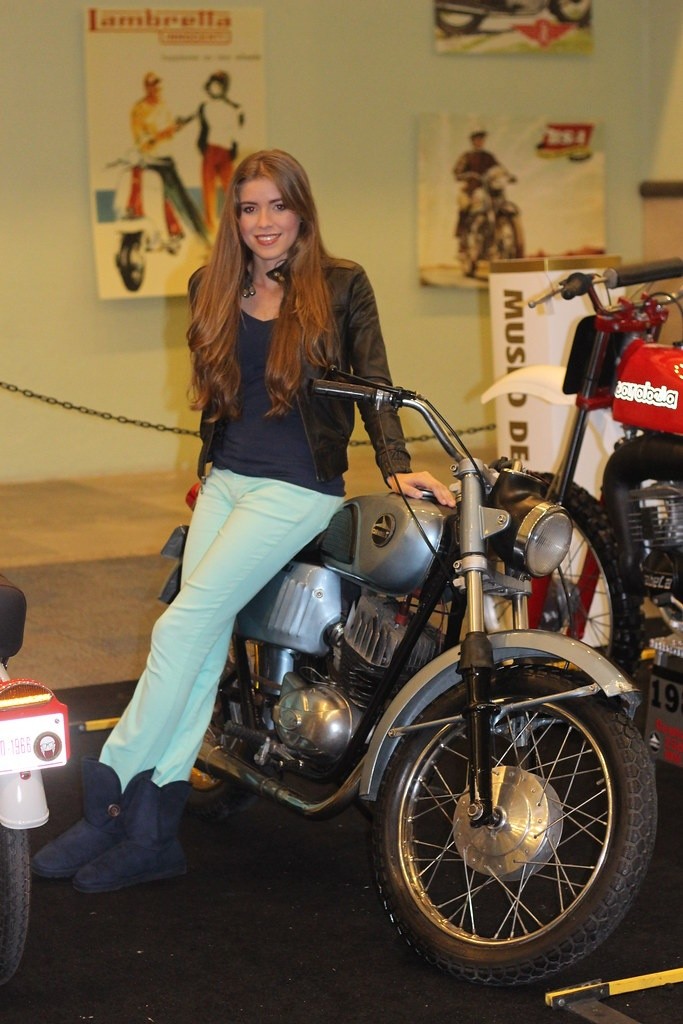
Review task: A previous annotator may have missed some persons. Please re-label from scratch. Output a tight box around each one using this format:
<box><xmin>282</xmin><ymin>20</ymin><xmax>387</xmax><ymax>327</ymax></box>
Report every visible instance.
<box><xmin>27</xmin><ymin>148</ymin><xmax>458</xmax><ymax>896</ymax></box>
<box><xmin>125</xmin><ymin>69</ymin><xmax>245</xmax><ymax>237</ymax></box>
<box><xmin>454</xmin><ymin>131</ymin><xmax>518</xmax><ymax>254</ymax></box>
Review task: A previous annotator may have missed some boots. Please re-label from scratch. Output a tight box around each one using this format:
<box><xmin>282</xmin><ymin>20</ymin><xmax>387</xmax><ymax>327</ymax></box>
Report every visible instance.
<box><xmin>72</xmin><ymin>780</ymin><xmax>194</xmax><ymax>894</ymax></box>
<box><xmin>31</xmin><ymin>756</ymin><xmax>155</xmax><ymax>879</ymax></box>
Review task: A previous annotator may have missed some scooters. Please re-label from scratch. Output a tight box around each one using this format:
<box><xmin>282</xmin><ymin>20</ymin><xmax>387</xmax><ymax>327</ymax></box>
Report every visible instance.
<box><xmin>105</xmin><ymin>145</ymin><xmax>184</xmax><ymax>293</ymax></box>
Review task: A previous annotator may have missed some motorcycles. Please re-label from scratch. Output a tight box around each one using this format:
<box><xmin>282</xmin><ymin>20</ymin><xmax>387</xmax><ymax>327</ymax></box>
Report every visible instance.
<box><xmin>480</xmin><ymin>256</ymin><xmax>683</xmax><ymax>773</ymax></box>
<box><xmin>453</xmin><ymin>173</ymin><xmax>522</xmax><ymax>280</ymax></box>
<box><xmin>155</xmin><ymin>357</ymin><xmax>658</xmax><ymax>993</ymax></box>
<box><xmin>1</xmin><ymin>578</ymin><xmax>71</xmax><ymax>1002</ymax></box>
<box><xmin>432</xmin><ymin>0</ymin><xmax>596</xmax><ymax>35</ymax></box>
